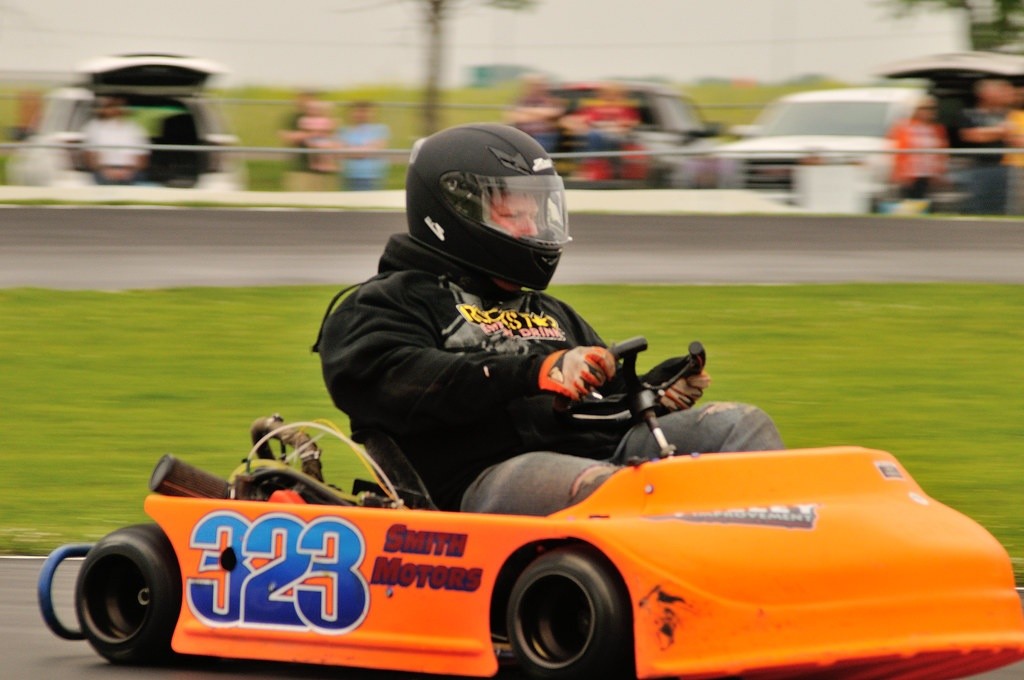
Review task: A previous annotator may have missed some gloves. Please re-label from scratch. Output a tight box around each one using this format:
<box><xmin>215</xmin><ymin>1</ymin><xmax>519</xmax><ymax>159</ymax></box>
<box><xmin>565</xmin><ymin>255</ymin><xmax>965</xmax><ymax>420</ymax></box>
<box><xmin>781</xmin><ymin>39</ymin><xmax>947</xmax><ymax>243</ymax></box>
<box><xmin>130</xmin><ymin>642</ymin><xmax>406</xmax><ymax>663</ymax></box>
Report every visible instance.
<box><xmin>523</xmin><ymin>345</ymin><xmax>616</xmax><ymax>408</ymax></box>
<box><xmin>650</xmin><ymin>356</ymin><xmax>711</xmax><ymax>418</ymax></box>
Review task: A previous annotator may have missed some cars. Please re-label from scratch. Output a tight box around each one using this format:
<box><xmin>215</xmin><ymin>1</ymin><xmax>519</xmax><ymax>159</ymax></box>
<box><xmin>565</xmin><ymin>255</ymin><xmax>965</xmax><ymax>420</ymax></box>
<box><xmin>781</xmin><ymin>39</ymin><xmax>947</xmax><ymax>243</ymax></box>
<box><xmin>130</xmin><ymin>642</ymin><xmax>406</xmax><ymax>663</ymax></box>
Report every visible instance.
<box><xmin>718</xmin><ymin>89</ymin><xmax>964</xmax><ymax>211</ymax></box>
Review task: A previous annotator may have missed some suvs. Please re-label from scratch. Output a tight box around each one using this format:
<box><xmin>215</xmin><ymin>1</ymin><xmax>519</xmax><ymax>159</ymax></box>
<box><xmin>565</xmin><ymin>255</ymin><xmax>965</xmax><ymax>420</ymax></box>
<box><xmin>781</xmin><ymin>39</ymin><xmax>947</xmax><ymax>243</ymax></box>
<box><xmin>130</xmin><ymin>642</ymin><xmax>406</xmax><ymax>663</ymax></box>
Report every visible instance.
<box><xmin>0</xmin><ymin>48</ymin><xmax>241</xmax><ymax>197</ymax></box>
<box><xmin>881</xmin><ymin>49</ymin><xmax>1024</xmax><ymax>207</ymax></box>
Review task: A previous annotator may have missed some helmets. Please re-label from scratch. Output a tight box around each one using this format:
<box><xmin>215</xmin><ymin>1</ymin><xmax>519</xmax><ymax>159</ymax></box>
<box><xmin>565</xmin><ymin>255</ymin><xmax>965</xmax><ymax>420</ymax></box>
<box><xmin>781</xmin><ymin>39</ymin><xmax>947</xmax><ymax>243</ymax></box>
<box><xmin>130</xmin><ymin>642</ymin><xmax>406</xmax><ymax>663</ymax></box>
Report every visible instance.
<box><xmin>405</xmin><ymin>120</ymin><xmax>573</xmax><ymax>291</ymax></box>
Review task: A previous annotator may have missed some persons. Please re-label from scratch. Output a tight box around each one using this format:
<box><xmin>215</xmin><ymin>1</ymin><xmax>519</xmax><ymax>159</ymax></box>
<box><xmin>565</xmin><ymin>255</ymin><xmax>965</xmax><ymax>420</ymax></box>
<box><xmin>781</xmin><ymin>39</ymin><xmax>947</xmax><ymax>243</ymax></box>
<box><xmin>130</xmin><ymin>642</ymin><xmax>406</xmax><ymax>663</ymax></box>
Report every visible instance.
<box><xmin>310</xmin><ymin>122</ymin><xmax>787</xmax><ymax>517</ymax></box>
<box><xmin>507</xmin><ymin>72</ymin><xmax>651</xmax><ymax>181</ymax></box>
<box><xmin>276</xmin><ymin>90</ymin><xmax>390</xmax><ymax>191</ymax></box>
<box><xmin>76</xmin><ymin>93</ymin><xmax>151</xmax><ymax>187</ymax></box>
<box><xmin>884</xmin><ymin>78</ymin><xmax>1024</xmax><ymax>214</ymax></box>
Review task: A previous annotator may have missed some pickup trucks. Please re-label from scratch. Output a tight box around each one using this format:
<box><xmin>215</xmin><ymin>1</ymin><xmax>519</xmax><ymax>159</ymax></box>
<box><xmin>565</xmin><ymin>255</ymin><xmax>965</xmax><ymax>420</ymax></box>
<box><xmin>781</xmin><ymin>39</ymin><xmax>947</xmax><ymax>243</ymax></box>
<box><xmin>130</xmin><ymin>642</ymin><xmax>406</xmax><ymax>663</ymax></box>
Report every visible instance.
<box><xmin>526</xmin><ymin>83</ymin><xmax>744</xmax><ymax>192</ymax></box>
